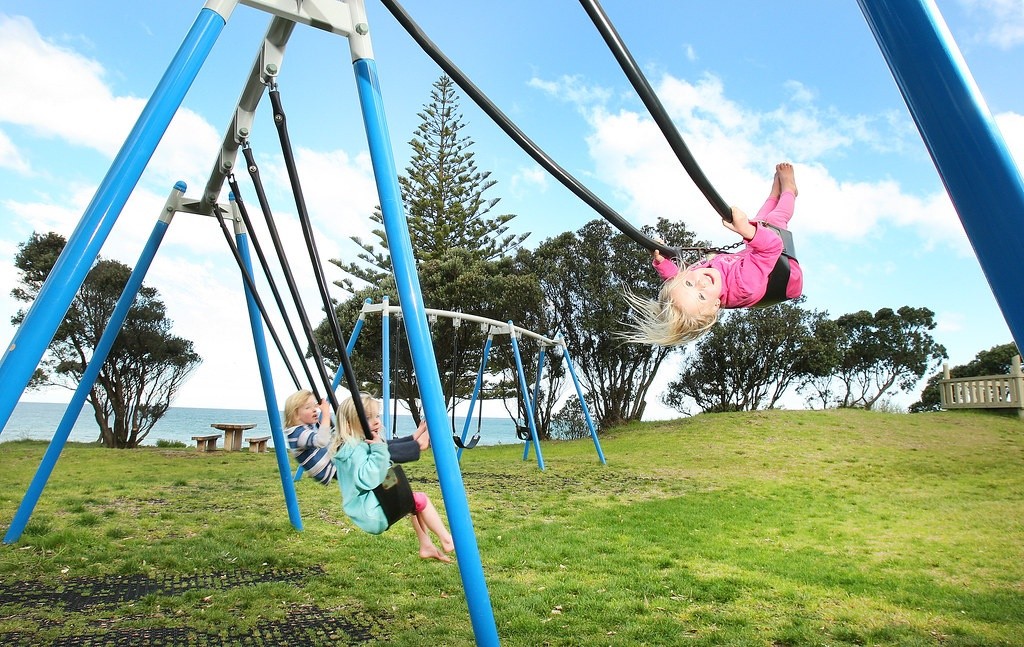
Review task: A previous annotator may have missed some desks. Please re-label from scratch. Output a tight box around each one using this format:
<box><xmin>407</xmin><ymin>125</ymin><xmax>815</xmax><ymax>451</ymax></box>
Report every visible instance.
<box><xmin>210</xmin><ymin>422</ymin><xmax>257</xmax><ymax>452</ymax></box>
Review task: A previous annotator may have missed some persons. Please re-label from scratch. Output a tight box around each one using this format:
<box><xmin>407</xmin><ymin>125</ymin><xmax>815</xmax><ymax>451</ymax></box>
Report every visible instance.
<box><xmin>284</xmin><ymin>389</ymin><xmax>429</xmax><ymax>487</ymax></box>
<box><xmin>609</xmin><ymin>161</ymin><xmax>803</xmax><ymax>349</ymax></box>
<box><xmin>329</xmin><ymin>391</ymin><xmax>454</xmax><ymax>563</ymax></box>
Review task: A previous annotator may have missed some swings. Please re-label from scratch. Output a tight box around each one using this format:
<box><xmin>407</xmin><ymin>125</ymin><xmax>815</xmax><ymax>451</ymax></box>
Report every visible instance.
<box><xmin>449</xmin><ymin>319</ymin><xmax>490</xmax><ymax>451</ymax></box>
<box><xmin>211</xmin><ymin>176</ymin><xmax>341</xmax><ymax>426</ymax></box>
<box><xmin>242</xmin><ymin>87</ymin><xmax>418</xmax><ymax>531</ymax></box>
<box><xmin>516</xmin><ymin>336</ymin><xmax>547</xmax><ymax>441</ymax></box>
<box><xmin>381</xmin><ymin>0</ymin><xmax>800</xmax><ymax>309</ymax></box>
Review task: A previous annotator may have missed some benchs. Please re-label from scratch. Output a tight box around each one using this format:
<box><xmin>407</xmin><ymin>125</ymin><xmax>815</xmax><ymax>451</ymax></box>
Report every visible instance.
<box><xmin>191</xmin><ymin>433</ymin><xmax>223</xmax><ymax>452</ymax></box>
<box><xmin>245</xmin><ymin>435</ymin><xmax>273</xmax><ymax>452</ymax></box>
